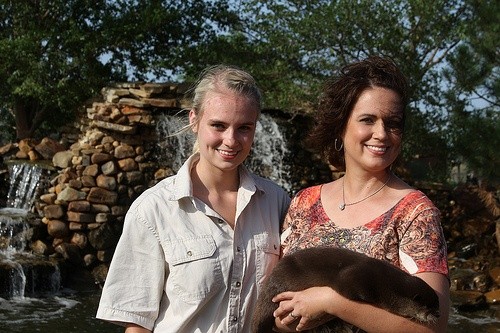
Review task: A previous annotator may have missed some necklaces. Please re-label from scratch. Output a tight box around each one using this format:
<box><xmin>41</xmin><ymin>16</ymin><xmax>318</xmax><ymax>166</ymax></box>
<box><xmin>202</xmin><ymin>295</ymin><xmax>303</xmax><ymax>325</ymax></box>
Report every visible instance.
<box><xmin>339</xmin><ymin>174</ymin><xmax>392</xmax><ymax>211</ymax></box>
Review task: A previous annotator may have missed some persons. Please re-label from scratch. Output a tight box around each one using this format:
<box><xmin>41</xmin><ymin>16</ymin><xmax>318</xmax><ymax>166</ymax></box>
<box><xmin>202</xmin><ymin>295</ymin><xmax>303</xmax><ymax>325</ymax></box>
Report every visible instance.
<box><xmin>272</xmin><ymin>57</ymin><xmax>452</xmax><ymax>333</ymax></box>
<box><xmin>95</xmin><ymin>65</ymin><xmax>292</xmax><ymax>333</ymax></box>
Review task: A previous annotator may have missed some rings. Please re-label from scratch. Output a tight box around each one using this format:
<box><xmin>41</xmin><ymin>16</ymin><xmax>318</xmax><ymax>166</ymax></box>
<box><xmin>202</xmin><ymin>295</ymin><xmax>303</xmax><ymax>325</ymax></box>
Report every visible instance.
<box><xmin>289</xmin><ymin>311</ymin><xmax>299</xmax><ymax>320</ymax></box>
